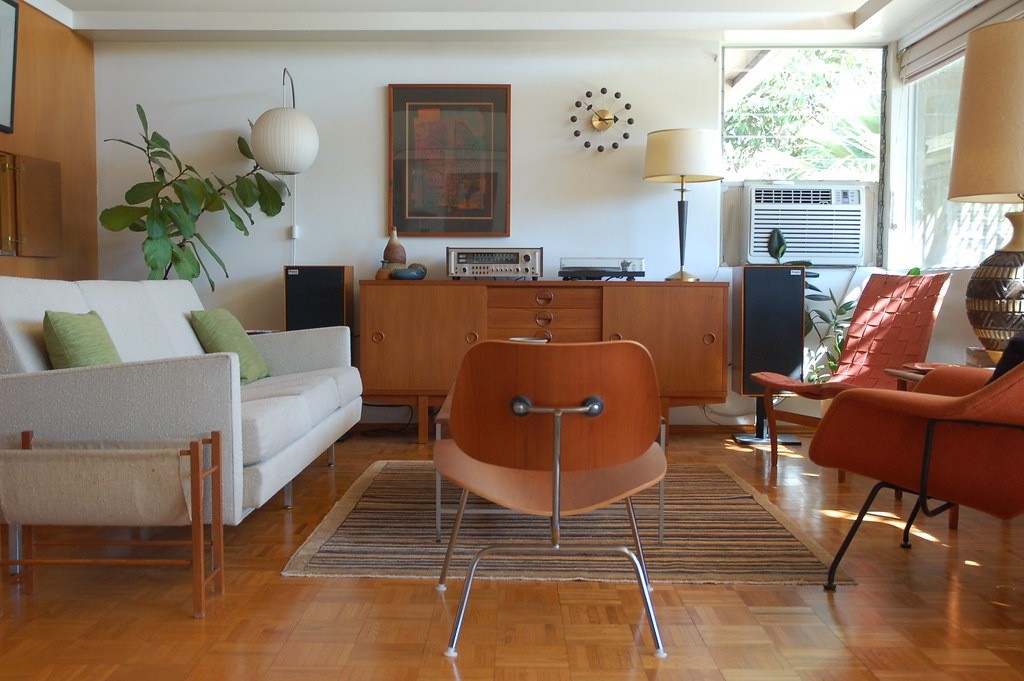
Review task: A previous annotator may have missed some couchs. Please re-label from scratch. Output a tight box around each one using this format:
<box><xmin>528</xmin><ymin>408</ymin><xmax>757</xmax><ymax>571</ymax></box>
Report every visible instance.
<box><xmin>0</xmin><ymin>276</ymin><xmax>365</xmax><ymax>569</ymax></box>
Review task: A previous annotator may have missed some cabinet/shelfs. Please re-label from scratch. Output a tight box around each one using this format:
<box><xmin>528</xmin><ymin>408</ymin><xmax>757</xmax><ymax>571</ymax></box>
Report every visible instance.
<box><xmin>354</xmin><ymin>278</ymin><xmax>734</xmax><ymax>447</ymax></box>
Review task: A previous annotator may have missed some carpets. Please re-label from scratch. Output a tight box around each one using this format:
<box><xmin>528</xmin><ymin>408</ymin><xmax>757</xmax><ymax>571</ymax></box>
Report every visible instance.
<box><xmin>279</xmin><ymin>452</ymin><xmax>857</xmax><ymax>581</ymax></box>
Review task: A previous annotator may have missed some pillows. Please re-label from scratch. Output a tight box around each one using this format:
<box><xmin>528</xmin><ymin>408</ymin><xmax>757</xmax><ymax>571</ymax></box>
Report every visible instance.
<box><xmin>43</xmin><ymin>309</ymin><xmax>124</xmax><ymax>369</ymax></box>
<box><xmin>189</xmin><ymin>308</ymin><xmax>271</xmax><ymax>385</ymax></box>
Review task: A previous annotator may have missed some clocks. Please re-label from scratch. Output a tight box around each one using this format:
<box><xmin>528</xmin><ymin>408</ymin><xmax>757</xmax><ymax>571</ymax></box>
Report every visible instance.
<box><xmin>569</xmin><ymin>88</ymin><xmax>634</xmax><ymax>152</ymax></box>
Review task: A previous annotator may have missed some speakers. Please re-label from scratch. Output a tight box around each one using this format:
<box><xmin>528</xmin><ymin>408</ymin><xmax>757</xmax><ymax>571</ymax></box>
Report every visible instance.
<box><xmin>732</xmin><ymin>264</ymin><xmax>805</xmax><ymax>396</ymax></box>
<box><xmin>282</xmin><ymin>265</ymin><xmax>354</xmax><ymax>346</ymax></box>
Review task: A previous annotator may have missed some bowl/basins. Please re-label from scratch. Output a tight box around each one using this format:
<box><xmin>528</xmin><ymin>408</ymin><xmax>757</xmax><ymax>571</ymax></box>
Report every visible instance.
<box><xmin>393</xmin><ymin>269</ymin><xmax>426</xmax><ymax>280</ymax></box>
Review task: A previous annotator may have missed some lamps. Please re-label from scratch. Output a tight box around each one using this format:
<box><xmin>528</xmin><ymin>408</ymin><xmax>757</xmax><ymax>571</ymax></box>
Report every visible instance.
<box><xmin>644</xmin><ymin>126</ymin><xmax>726</xmax><ymax>282</ymax></box>
<box><xmin>945</xmin><ymin>19</ymin><xmax>1024</xmax><ymax>367</ymax></box>
<box><xmin>248</xmin><ymin>66</ymin><xmax>321</xmax><ymax>262</ymax></box>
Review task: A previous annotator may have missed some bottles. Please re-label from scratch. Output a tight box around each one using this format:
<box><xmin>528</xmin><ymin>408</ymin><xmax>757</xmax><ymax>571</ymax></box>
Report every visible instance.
<box><xmin>375</xmin><ymin>260</ymin><xmax>392</xmax><ymax>280</ymax></box>
<box><xmin>383</xmin><ymin>226</ymin><xmax>406</xmax><ymax>269</ymax></box>
<box><xmin>408</xmin><ymin>263</ymin><xmax>427</xmax><ymax>275</ymax></box>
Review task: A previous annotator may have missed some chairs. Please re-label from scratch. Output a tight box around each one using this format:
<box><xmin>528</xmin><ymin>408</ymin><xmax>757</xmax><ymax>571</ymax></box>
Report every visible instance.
<box><xmin>430</xmin><ymin>336</ymin><xmax>673</xmax><ymax>662</ymax></box>
<box><xmin>747</xmin><ymin>275</ymin><xmax>954</xmax><ymax>484</ymax></box>
<box><xmin>806</xmin><ymin>331</ymin><xmax>1024</xmax><ymax>596</ymax></box>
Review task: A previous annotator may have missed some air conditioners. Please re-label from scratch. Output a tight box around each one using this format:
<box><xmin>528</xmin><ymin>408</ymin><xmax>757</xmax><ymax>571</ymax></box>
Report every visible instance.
<box><xmin>737</xmin><ymin>180</ymin><xmax>866</xmax><ymax>265</ymax></box>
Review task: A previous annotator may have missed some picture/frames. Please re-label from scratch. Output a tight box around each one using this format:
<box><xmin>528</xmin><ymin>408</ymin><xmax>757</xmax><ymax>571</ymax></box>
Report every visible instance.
<box><xmin>387</xmin><ymin>82</ymin><xmax>512</xmax><ymax>238</ymax></box>
<box><xmin>0</xmin><ymin>0</ymin><xmax>21</xmax><ymax>136</ymax></box>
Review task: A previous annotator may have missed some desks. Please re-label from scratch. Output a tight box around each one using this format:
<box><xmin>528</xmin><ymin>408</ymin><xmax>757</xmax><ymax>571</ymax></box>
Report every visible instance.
<box><xmin>434</xmin><ymin>368</ymin><xmax>666</xmax><ymax>547</ymax></box>
<box><xmin>881</xmin><ymin>364</ymin><xmax>973</xmax><ymax>533</ymax></box>
